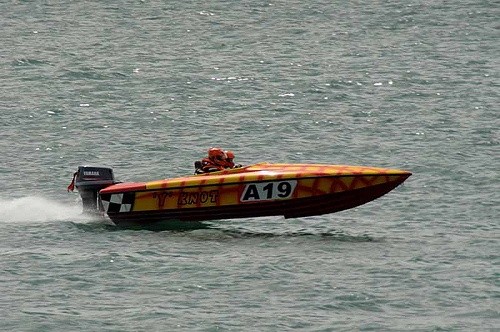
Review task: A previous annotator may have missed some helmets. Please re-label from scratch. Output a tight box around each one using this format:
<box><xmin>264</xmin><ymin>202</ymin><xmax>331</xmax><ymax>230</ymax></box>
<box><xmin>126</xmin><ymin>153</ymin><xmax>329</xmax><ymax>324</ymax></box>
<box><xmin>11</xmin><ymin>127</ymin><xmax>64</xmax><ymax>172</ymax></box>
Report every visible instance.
<box><xmin>223</xmin><ymin>150</ymin><xmax>235</xmax><ymax>167</ymax></box>
<box><xmin>208</xmin><ymin>147</ymin><xmax>225</xmax><ymax>164</ymax></box>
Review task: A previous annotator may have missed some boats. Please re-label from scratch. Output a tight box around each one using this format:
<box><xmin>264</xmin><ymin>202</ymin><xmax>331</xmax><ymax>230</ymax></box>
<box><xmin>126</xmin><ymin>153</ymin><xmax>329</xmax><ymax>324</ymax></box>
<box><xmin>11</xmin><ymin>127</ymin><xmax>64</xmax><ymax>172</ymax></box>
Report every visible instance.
<box><xmin>70</xmin><ymin>162</ymin><xmax>412</xmax><ymax>226</ymax></box>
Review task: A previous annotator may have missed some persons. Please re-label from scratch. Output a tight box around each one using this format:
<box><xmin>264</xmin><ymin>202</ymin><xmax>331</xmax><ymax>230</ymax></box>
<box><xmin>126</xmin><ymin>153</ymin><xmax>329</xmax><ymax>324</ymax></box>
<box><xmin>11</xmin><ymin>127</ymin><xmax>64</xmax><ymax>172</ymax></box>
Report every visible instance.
<box><xmin>223</xmin><ymin>150</ymin><xmax>242</xmax><ymax>169</ymax></box>
<box><xmin>201</xmin><ymin>147</ymin><xmax>227</xmax><ymax>173</ymax></box>
